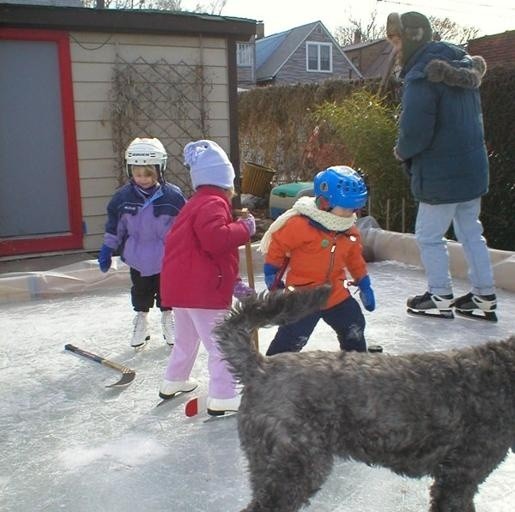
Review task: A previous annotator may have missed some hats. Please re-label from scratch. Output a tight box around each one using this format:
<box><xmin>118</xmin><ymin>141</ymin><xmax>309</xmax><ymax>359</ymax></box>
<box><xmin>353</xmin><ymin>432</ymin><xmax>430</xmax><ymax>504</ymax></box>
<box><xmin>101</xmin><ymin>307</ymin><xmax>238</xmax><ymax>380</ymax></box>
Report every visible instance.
<box><xmin>386</xmin><ymin>10</ymin><xmax>433</xmax><ymax>67</ymax></box>
<box><xmin>183</xmin><ymin>138</ymin><xmax>236</xmax><ymax>191</ymax></box>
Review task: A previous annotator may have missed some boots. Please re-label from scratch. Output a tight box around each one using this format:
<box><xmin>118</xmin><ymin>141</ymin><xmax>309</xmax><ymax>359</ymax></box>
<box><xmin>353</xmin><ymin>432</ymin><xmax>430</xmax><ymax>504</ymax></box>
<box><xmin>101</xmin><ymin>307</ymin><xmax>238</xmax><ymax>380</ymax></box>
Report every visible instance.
<box><xmin>129</xmin><ymin>311</ymin><xmax>151</xmax><ymax>348</ymax></box>
<box><xmin>453</xmin><ymin>290</ymin><xmax>500</xmax><ymax>311</ymax></box>
<box><xmin>406</xmin><ymin>291</ymin><xmax>456</xmax><ymax>311</ymax></box>
<box><xmin>161</xmin><ymin>311</ymin><xmax>176</xmax><ymax>346</ymax></box>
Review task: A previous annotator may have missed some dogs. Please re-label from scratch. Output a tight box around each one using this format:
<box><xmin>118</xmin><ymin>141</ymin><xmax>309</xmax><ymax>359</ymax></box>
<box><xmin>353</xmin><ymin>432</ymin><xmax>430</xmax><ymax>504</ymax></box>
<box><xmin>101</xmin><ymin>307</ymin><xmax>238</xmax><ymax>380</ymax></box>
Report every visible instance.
<box><xmin>210</xmin><ymin>278</ymin><xmax>515</xmax><ymax>512</ymax></box>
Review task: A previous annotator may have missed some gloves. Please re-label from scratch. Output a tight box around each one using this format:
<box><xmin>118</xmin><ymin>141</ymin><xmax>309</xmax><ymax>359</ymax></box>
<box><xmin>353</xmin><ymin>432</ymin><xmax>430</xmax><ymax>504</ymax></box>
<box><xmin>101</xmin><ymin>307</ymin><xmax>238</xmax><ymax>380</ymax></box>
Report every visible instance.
<box><xmin>358</xmin><ymin>275</ymin><xmax>376</xmax><ymax>313</ymax></box>
<box><xmin>264</xmin><ymin>263</ymin><xmax>283</xmax><ymax>290</ymax></box>
<box><xmin>238</xmin><ymin>214</ymin><xmax>258</xmax><ymax>237</ymax></box>
<box><xmin>97</xmin><ymin>243</ymin><xmax>113</xmax><ymax>273</ymax></box>
<box><xmin>233</xmin><ymin>279</ymin><xmax>256</xmax><ymax>303</ymax></box>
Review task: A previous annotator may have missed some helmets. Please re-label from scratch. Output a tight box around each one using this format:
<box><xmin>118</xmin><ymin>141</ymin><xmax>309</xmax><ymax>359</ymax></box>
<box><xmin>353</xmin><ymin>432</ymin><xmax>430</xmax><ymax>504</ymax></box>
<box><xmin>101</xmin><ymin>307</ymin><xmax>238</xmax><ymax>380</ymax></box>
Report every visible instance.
<box><xmin>124</xmin><ymin>135</ymin><xmax>168</xmax><ymax>182</ymax></box>
<box><xmin>312</xmin><ymin>164</ymin><xmax>373</xmax><ymax>214</ymax></box>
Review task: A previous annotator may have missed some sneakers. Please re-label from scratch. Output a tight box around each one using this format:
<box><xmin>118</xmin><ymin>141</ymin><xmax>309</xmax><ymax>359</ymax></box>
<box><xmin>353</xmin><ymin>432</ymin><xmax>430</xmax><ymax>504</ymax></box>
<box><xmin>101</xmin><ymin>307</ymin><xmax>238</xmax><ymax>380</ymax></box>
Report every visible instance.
<box><xmin>205</xmin><ymin>394</ymin><xmax>243</xmax><ymax>416</ymax></box>
<box><xmin>157</xmin><ymin>376</ymin><xmax>201</xmax><ymax>398</ymax></box>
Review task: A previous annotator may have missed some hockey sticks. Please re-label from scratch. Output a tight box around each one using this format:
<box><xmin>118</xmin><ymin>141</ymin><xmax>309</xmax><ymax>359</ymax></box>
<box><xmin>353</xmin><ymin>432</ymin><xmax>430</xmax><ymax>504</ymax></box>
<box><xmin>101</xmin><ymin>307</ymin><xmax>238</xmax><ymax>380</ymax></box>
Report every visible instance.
<box><xmin>65</xmin><ymin>344</ymin><xmax>135</xmax><ymax>387</ymax></box>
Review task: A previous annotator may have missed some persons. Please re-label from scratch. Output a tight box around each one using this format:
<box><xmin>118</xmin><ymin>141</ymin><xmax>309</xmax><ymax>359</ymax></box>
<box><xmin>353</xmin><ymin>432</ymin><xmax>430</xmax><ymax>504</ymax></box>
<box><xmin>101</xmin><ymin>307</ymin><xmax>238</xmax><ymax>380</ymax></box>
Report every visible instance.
<box><xmin>385</xmin><ymin>8</ymin><xmax>499</xmax><ymax>313</ymax></box>
<box><xmin>155</xmin><ymin>140</ymin><xmax>259</xmax><ymax>419</ymax></box>
<box><xmin>96</xmin><ymin>136</ymin><xmax>187</xmax><ymax>347</ymax></box>
<box><xmin>262</xmin><ymin>164</ymin><xmax>377</xmax><ymax>356</ymax></box>
